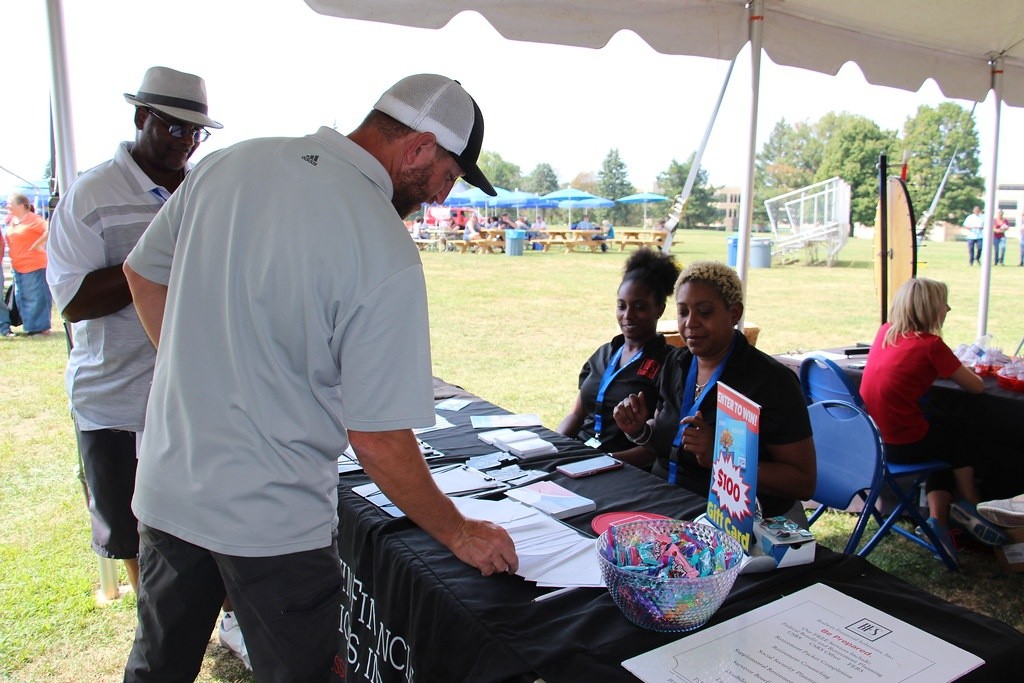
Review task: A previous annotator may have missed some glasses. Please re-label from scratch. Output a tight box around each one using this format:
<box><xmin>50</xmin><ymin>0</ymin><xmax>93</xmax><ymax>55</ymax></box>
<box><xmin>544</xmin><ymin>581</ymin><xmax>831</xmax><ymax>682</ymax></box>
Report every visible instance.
<box><xmin>144</xmin><ymin>107</ymin><xmax>212</xmax><ymax>143</ymax></box>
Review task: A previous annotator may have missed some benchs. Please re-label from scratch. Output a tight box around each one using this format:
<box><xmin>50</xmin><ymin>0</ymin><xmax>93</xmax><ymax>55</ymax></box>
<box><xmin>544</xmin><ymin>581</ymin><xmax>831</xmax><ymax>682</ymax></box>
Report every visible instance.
<box><xmin>413</xmin><ymin>240</ymin><xmax>665</xmax><ymax>245</ymax></box>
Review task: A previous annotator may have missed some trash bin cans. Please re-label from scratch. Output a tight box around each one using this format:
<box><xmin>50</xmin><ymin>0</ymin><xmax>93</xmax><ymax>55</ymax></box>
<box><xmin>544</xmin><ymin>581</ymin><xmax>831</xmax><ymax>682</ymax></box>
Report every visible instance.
<box><xmin>505</xmin><ymin>229</ymin><xmax>526</xmax><ymax>256</ymax></box>
<box><xmin>725</xmin><ymin>234</ymin><xmax>739</xmax><ymax>267</ymax></box>
<box><xmin>749</xmin><ymin>236</ymin><xmax>777</xmax><ymax>268</ymax></box>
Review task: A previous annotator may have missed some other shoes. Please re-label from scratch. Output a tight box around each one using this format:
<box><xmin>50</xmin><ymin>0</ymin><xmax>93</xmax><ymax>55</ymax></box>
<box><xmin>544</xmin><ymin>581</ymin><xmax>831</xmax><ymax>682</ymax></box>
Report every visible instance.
<box><xmin>977</xmin><ymin>493</ymin><xmax>1024</xmax><ymax>528</ymax></box>
<box><xmin>219</xmin><ymin>618</ymin><xmax>252</xmax><ymax>670</ymax></box>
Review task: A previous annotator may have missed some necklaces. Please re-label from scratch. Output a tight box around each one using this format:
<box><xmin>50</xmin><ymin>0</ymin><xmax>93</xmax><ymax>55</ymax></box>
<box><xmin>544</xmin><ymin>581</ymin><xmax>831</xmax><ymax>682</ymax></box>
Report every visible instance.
<box><xmin>694</xmin><ymin>380</ymin><xmax>708</xmax><ymax>400</ymax></box>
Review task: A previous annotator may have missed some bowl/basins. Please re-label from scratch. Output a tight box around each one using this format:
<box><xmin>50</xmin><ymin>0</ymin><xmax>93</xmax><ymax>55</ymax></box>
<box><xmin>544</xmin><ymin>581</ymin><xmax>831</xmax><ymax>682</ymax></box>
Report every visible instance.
<box><xmin>596</xmin><ymin>519</ymin><xmax>744</xmax><ymax>633</ymax></box>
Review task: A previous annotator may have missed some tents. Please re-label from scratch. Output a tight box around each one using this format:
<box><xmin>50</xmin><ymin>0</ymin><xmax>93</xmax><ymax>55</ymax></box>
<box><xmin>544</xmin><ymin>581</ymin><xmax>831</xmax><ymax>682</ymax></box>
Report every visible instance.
<box><xmin>46</xmin><ymin>0</ymin><xmax>1023</xmax><ymax>346</ymax></box>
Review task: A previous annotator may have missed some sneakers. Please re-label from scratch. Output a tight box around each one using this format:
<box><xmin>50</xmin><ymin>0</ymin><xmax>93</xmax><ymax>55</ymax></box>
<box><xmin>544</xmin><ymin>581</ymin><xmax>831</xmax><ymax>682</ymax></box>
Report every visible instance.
<box><xmin>914</xmin><ymin>518</ymin><xmax>961</xmax><ymax>569</ymax></box>
<box><xmin>950</xmin><ymin>500</ymin><xmax>1007</xmax><ymax>545</ymax></box>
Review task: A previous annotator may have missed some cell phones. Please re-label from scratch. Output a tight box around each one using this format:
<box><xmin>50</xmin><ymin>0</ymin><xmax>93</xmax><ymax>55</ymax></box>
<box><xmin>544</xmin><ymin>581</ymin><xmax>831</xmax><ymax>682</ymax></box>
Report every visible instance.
<box><xmin>556</xmin><ymin>455</ymin><xmax>623</xmax><ymax>478</ymax></box>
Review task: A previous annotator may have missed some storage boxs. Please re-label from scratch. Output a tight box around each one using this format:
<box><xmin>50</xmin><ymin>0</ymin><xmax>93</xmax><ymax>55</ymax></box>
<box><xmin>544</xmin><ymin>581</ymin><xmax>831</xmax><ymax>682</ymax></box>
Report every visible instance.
<box><xmin>752</xmin><ymin>515</ymin><xmax>816</xmax><ymax>567</ymax></box>
<box><xmin>994</xmin><ymin>527</ymin><xmax>1024</xmax><ymax>572</ymax></box>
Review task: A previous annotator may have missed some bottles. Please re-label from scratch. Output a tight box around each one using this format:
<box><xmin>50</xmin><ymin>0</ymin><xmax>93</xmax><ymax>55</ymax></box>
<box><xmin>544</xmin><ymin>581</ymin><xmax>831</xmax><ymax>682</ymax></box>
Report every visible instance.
<box><xmin>959</xmin><ymin>335</ymin><xmax>992</xmax><ymax>364</ymax></box>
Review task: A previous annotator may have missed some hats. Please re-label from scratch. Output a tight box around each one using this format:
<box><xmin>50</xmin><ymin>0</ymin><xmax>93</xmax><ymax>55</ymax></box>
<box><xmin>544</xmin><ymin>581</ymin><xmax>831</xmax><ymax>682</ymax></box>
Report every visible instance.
<box><xmin>375</xmin><ymin>73</ymin><xmax>499</xmax><ymax>198</ymax></box>
<box><xmin>123</xmin><ymin>67</ymin><xmax>224</xmax><ymax>129</ymax></box>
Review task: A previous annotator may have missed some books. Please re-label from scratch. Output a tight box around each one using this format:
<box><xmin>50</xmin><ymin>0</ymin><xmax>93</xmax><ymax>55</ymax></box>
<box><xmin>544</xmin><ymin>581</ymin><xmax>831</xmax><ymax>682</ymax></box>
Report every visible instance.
<box><xmin>470</xmin><ymin>414</ymin><xmax>543</xmax><ymax>430</ymax></box>
<box><xmin>477</xmin><ymin>427</ymin><xmax>558</xmax><ymax>459</ymax></box>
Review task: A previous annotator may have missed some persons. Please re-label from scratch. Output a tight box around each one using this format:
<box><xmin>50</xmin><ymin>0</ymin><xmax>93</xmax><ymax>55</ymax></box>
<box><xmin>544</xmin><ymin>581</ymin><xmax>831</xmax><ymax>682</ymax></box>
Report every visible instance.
<box><xmin>977</xmin><ymin>492</ymin><xmax>1023</xmax><ymax>530</ymax></box>
<box><xmin>571</xmin><ymin>215</ymin><xmax>615</xmax><ymax>252</ymax></box>
<box><xmin>0</xmin><ymin>191</ymin><xmax>53</xmax><ymax>339</ymax></box>
<box><xmin>413</xmin><ymin>211</ymin><xmax>549</xmax><ymax>254</ymax></box>
<box><xmin>963</xmin><ymin>206</ymin><xmax>985</xmax><ymax>266</ymax></box>
<box><xmin>1019</xmin><ymin>213</ymin><xmax>1024</xmax><ymax>267</ymax></box>
<box><xmin>859</xmin><ymin>276</ymin><xmax>1008</xmax><ymax>560</ymax></box>
<box><xmin>993</xmin><ymin>210</ymin><xmax>1009</xmax><ymax>267</ymax></box>
<box><xmin>42</xmin><ymin>63</ymin><xmax>259</xmax><ymax>673</ymax></box>
<box><xmin>555</xmin><ymin>247</ymin><xmax>818</xmax><ymax>531</ymax></box>
<box><xmin>125</xmin><ymin>72</ymin><xmax>519</xmax><ymax>682</ymax></box>
<box><xmin>654</xmin><ymin>218</ymin><xmax>667</xmax><ymax>251</ymax></box>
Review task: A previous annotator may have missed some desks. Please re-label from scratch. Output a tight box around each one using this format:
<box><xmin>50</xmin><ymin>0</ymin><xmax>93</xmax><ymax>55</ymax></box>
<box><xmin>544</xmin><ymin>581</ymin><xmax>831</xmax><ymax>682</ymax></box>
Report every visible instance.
<box><xmin>540</xmin><ymin>230</ymin><xmax>612</xmax><ymax>253</ymax></box>
<box><xmin>330</xmin><ymin>344</ymin><xmax>1024</xmax><ymax>683</ymax></box>
<box><xmin>615</xmin><ymin>232</ymin><xmax>668</xmax><ymax>251</ymax></box>
<box><xmin>472</xmin><ymin>230</ymin><xmax>505</xmax><ymax>254</ymax></box>
<box><xmin>420</xmin><ymin>229</ymin><xmax>464</xmax><ymax>251</ymax></box>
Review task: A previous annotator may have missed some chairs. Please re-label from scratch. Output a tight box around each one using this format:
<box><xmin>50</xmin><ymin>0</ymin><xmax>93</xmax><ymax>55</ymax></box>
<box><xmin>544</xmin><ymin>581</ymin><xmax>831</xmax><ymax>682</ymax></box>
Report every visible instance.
<box><xmin>807</xmin><ymin>400</ymin><xmax>886</xmax><ymax>554</ymax></box>
<box><xmin>799</xmin><ymin>356</ymin><xmax>963</xmax><ymax>573</ymax></box>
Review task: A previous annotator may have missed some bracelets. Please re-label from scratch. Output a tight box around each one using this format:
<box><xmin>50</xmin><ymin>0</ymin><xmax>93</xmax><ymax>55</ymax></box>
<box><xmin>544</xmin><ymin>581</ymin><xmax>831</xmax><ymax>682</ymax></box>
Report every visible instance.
<box><xmin>624</xmin><ymin>423</ymin><xmax>653</xmax><ymax>446</ymax></box>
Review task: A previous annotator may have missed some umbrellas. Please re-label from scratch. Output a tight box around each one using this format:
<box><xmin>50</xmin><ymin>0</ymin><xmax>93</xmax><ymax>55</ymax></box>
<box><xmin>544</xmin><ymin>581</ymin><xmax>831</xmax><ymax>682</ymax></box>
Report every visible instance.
<box><xmin>420</xmin><ymin>182</ymin><xmax>615</xmax><ymax>229</ymax></box>
<box><xmin>617</xmin><ymin>192</ymin><xmax>668</xmax><ymax>229</ymax></box>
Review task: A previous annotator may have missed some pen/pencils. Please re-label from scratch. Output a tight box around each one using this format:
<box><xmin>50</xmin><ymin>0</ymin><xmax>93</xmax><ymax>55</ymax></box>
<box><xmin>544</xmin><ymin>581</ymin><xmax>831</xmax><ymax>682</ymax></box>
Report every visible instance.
<box><xmin>530</xmin><ymin>586</ymin><xmax>578</xmax><ymax>602</ymax></box>
